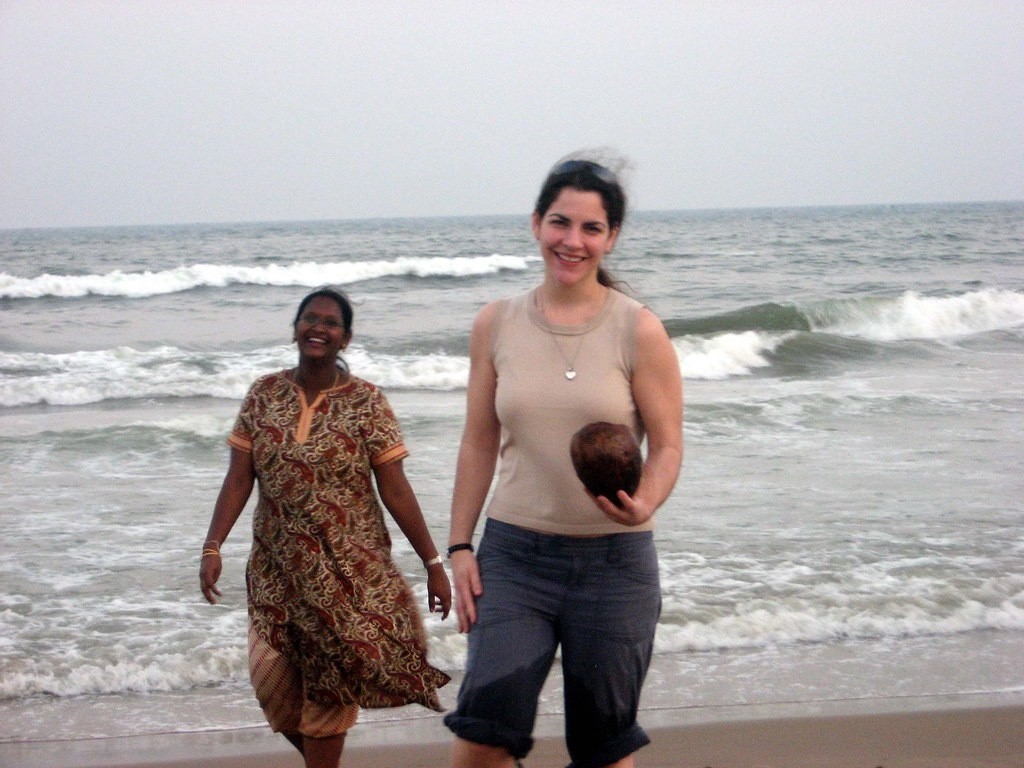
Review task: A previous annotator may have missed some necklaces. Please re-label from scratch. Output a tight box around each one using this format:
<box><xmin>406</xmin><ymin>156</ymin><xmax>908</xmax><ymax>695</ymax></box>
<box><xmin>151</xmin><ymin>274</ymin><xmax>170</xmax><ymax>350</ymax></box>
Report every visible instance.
<box><xmin>539</xmin><ymin>284</ymin><xmax>602</xmax><ymax>380</ymax></box>
<box><xmin>292</xmin><ymin>368</ymin><xmax>340</xmax><ymax>387</ymax></box>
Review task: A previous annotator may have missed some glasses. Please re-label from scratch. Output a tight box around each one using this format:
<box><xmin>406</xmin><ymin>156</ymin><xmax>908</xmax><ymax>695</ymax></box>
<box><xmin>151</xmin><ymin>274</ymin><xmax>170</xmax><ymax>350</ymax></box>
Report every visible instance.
<box><xmin>552</xmin><ymin>159</ymin><xmax>620</xmax><ymax>187</ymax></box>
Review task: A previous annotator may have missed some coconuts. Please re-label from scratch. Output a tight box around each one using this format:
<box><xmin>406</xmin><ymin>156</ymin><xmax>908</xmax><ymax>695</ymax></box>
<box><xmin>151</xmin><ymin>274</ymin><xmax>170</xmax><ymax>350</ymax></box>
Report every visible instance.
<box><xmin>570</xmin><ymin>422</ymin><xmax>642</xmax><ymax>506</ymax></box>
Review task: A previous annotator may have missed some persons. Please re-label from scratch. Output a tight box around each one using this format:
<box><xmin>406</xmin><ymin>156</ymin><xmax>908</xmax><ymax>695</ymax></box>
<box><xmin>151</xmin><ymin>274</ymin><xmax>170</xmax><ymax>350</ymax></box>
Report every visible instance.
<box><xmin>446</xmin><ymin>160</ymin><xmax>684</xmax><ymax>768</ymax></box>
<box><xmin>199</xmin><ymin>289</ymin><xmax>452</xmax><ymax>768</ymax></box>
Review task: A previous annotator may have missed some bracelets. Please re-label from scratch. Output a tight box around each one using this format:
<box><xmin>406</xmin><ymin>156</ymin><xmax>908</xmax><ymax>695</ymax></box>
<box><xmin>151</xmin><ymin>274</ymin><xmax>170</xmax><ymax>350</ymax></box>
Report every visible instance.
<box><xmin>201</xmin><ymin>539</ymin><xmax>221</xmax><ymax>557</ymax></box>
<box><xmin>446</xmin><ymin>543</ymin><xmax>474</xmax><ymax>559</ymax></box>
<box><xmin>424</xmin><ymin>555</ymin><xmax>443</xmax><ymax>568</ymax></box>
